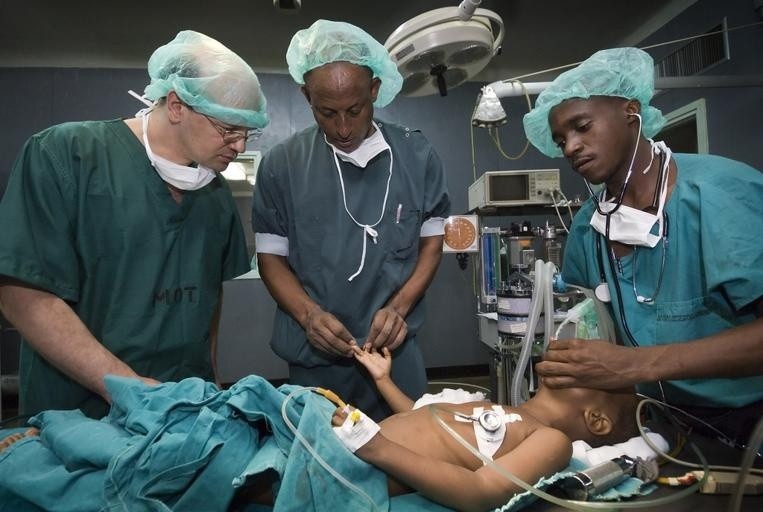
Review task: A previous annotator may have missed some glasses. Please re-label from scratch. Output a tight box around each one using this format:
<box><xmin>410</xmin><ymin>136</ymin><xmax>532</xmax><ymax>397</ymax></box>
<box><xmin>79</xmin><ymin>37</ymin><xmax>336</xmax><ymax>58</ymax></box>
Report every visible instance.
<box><xmin>204</xmin><ymin>115</ymin><xmax>262</xmax><ymax>143</ymax></box>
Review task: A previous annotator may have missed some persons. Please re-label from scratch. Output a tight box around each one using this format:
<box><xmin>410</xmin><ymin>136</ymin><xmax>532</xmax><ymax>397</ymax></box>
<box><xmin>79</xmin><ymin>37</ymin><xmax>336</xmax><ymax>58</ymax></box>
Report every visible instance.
<box><xmin>523</xmin><ymin>46</ymin><xmax>762</xmax><ymax>457</ymax></box>
<box><xmin>250</xmin><ymin>16</ymin><xmax>454</xmax><ymax>424</ymax></box>
<box><xmin>0</xmin><ymin>26</ymin><xmax>268</xmax><ymax>428</ymax></box>
<box><xmin>0</xmin><ymin>344</ymin><xmax>647</xmax><ymax>512</ymax></box>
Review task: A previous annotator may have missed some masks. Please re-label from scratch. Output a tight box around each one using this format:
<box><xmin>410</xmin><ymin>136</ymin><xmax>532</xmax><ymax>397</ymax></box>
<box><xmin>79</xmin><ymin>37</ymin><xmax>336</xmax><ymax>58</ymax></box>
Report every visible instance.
<box><xmin>143</xmin><ymin>119</ymin><xmax>216</xmax><ymax>191</ymax></box>
<box><xmin>323</xmin><ymin>121</ymin><xmax>394</xmax><ymax>169</ymax></box>
<box><xmin>590</xmin><ymin>192</ymin><xmax>667</xmax><ymax>249</ymax></box>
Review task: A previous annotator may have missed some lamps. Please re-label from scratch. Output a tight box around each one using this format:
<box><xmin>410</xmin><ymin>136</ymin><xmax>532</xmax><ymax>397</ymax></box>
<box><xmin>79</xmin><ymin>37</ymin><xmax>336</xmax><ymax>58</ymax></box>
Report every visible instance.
<box><xmin>379</xmin><ymin>0</ymin><xmax>511</xmax><ymax>102</ymax></box>
